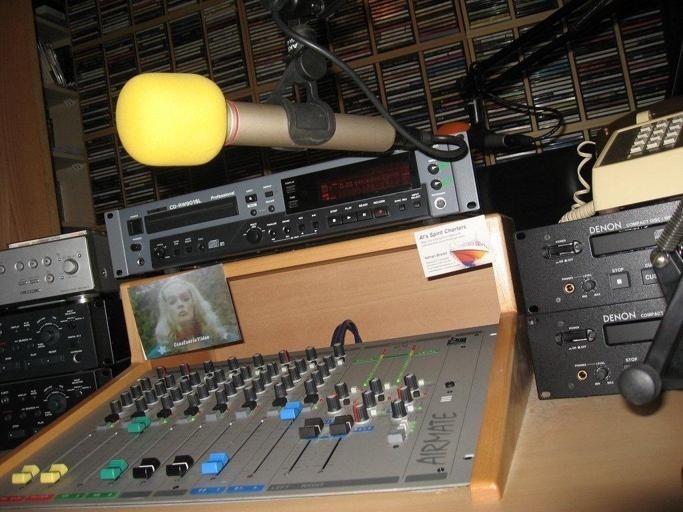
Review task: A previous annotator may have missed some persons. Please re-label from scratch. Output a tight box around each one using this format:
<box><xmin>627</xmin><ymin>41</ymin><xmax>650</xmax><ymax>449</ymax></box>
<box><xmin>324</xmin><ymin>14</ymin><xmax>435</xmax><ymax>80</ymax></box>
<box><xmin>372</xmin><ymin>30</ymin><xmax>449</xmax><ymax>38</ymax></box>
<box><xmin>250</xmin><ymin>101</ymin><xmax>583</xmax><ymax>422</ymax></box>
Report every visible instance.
<box><xmin>147</xmin><ymin>278</ymin><xmax>241</xmax><ymax>360</ymax></box>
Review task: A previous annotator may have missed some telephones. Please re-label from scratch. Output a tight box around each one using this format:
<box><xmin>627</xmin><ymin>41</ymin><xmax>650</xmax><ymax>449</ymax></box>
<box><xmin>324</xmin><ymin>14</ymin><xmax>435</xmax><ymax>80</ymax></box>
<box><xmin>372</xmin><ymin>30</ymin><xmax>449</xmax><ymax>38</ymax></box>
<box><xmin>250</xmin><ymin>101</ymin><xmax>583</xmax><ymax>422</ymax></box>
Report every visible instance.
<box><xmin>558</xmin><ymin>95</ymin><xmax>683</xmax><ymax>225</ymax></box>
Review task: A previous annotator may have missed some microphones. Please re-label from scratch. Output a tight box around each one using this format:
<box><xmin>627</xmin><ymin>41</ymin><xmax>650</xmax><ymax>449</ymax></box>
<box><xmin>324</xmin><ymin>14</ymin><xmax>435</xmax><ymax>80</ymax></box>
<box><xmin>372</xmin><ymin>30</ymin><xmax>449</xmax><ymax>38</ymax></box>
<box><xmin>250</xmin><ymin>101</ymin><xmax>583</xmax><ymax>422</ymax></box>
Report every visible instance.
<box><xmin>115</xmin><ymin>72</ymin><xmax>432</xmax><ymax>167</ymax></box>
<box><xmin>466</xmin><ymin>124</ymin><xmax>533</xmax><ymax>152</ymax></box>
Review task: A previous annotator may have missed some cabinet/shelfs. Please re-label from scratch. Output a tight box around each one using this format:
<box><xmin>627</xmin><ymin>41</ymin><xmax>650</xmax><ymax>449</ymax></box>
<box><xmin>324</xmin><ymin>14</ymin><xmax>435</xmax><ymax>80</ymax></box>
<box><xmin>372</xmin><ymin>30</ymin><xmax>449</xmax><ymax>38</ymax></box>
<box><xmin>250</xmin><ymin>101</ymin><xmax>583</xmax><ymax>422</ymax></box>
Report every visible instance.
<box><xmin>0</xmin><ymin>0</ymin><xmax>683</xmax><ymax>251</ymax></box>
